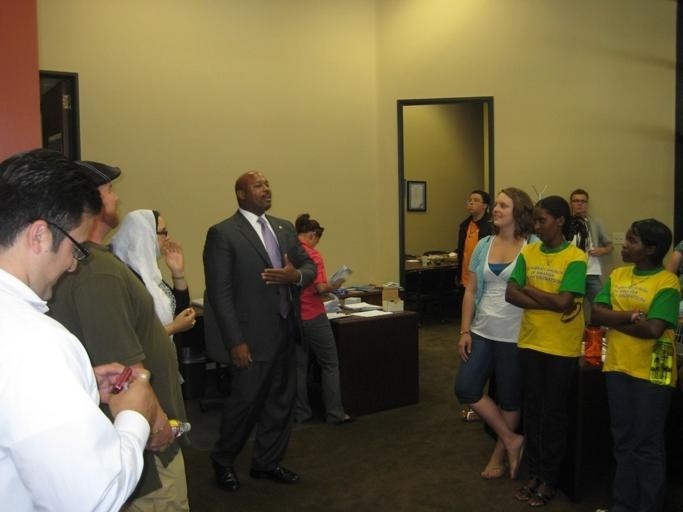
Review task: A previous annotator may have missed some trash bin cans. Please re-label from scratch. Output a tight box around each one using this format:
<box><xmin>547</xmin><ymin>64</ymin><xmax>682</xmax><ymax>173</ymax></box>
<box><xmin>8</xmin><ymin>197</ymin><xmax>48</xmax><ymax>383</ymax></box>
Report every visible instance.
<box><xmin>178</xmin><ymin>346</ymin><xmax>208</xmax><ymax>401</ymax></box>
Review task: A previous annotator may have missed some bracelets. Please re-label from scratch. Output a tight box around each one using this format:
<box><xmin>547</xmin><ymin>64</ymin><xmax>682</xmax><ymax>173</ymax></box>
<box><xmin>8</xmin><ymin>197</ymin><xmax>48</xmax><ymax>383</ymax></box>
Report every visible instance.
<box><xmin>149</xmin><ymin>412</ymin><xmax>168</xmax><ymax>439</ymax></box>
<box><xmin>170</xmin><ymin>275</ymin><xmax>185</xmax><ymax>280</ymax></box>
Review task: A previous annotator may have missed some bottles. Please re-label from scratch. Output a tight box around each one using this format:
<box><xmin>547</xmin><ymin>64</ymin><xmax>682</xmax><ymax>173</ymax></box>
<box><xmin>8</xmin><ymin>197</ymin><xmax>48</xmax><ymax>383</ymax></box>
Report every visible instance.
<box><xmin>649</xmin><ymin>337</ymin><xmax>673</xmax><ymax>384</ymax></box>
<box><xmin>167</xmin><ymin>419</ymin><xmax>191</xmax><ymax>440</ymax></box>
<box><xmin>583</xmin><ymin>323</ymin><xmax>603</xmax><ymax>367</ymax></box>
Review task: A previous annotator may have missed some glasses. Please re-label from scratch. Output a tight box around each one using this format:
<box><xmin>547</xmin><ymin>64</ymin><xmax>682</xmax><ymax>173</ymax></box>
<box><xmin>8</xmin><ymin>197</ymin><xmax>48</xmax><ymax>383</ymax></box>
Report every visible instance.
<box><xmin>157</xmin><ymin>231</ymin><xmax>167</xmax><ymax>236</ymax></box>
<box><xmin>30</xmin><ymin>218</ymin><xmax>90</xmax><ymax>261</ymax></box>
<box><xmin>571</xmin><ymin>199</ymin><xmax>588</xmax><ymax>203</ymax></box>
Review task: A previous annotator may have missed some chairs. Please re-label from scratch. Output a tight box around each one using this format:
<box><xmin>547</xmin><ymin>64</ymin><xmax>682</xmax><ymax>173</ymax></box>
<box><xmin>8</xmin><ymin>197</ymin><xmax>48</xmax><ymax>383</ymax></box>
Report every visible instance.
<box><xmin>405</xmin><ymin>249</ymin><xmax>463</xmax><ymax>328</ymax></box>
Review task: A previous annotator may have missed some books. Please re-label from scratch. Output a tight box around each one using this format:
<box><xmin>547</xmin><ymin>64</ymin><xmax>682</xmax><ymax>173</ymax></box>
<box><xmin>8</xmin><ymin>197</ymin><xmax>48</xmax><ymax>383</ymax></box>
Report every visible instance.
<box><xmin>329</xmin><ymin>264</ymin><xmax>354</xmax><ymax>286</ymax></box>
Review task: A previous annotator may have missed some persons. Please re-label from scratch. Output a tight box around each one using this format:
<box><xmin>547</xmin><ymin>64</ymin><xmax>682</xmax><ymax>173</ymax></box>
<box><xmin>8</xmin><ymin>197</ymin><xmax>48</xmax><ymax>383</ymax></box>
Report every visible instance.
<box><xmin>44</xmin><ymin>157</ymin><xmax>191</xmax><ymax>511</ymax></box>
<box><xmin>456</xmin><ymin>187</ymin><xmax>614</xmax><ymax>507</ymax></box>
<box><xmin>591</xmin><ymin>219</ymin><xmax>682</xmax><ymax>510</ymax></box>
<box><xmin>201</xmin><ymin>170</ymin><xmax>316</xmax><ymax>492</ymax></box>
<box><xmin>0</xmin><ymin>148</ymin><xmax>160</xmax><ymax>512</ymax></box>
<box><xmin>110</xmin><ymin>206</ymin><xmax>198</xmax><ymax>388</ymax></box>
<box><xmin>293</xmin><ymin>212</ymin><xmax>357</xmax><ymax>427</ymax></box>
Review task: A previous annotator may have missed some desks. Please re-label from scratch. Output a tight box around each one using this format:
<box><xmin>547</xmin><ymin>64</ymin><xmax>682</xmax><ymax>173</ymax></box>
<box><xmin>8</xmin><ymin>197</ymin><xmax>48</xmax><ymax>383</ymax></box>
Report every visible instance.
<box><xmin>173</xmin><ymin>284</ymin><xmax>417</xmax><ymax>419</ymax></box>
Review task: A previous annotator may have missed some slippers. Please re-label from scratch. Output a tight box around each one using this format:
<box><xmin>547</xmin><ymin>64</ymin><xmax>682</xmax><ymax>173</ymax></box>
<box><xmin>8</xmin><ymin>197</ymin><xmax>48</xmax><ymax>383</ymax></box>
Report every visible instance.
<box><xmin>481</xmin><ymin>437</ymin><xmax>527</xmax><ymax>479</ymax></box>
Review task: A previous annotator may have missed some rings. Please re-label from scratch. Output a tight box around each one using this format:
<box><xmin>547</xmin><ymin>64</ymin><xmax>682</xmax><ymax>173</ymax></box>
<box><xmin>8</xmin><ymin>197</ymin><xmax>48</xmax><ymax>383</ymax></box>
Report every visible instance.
<box><xmin>191</xmin><ymin>320</ymin><xmax>197</xmax><ymax>325</ymax></box>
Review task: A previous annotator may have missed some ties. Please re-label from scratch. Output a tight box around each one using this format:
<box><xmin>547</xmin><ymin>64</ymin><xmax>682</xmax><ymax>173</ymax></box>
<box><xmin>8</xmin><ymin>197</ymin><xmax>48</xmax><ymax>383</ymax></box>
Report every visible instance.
<box><xmin>258</xmin><ymin>217</ymin><xmax>292</xmax><ymax>319</ymax></box>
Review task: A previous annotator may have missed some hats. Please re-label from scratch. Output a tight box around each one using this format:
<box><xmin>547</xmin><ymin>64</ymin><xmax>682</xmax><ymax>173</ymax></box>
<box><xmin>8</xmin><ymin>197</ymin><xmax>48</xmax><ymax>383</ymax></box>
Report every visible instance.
<box><xmin>74</xmin><ymin>160</ymin><xmax>121</xmax><ymax>187</ymax></box>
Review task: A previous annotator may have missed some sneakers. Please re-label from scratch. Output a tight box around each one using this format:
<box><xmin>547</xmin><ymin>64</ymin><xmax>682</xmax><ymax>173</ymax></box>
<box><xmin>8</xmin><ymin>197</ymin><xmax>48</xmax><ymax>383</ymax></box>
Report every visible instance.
<box><xmin>343</xmin><ymin>412</ymin><xmax>356</xmax><ymax>423</ymax></box>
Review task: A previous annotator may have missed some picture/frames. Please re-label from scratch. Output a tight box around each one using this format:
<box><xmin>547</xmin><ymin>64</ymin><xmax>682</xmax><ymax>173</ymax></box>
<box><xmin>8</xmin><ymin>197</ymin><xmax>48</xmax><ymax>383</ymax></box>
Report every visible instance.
<box><xmin>407</xmin><ymin>180</ymin><xmax>427</xmax><ymax>211</ymax></box>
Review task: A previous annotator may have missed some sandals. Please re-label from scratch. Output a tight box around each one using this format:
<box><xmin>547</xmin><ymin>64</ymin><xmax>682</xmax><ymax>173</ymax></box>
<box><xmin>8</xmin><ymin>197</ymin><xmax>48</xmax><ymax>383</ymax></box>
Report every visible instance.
<box><xmin>515</xmin><ymin>476</ymin><xmax>560</xmax><ymax>506</ymax></box>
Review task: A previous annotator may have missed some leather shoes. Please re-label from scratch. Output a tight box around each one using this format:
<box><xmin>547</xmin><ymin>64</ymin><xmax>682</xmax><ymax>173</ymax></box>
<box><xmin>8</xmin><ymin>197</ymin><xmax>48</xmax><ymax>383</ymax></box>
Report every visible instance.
<box><xmin>211</xmin><ymin>457</ymin><xmax>240</xmax><ymax>492</ymax></box>
<box><xmin>250</xmin><ymin>465</ymin><xmax>300</xmax><ymax>484</ymax></box>
